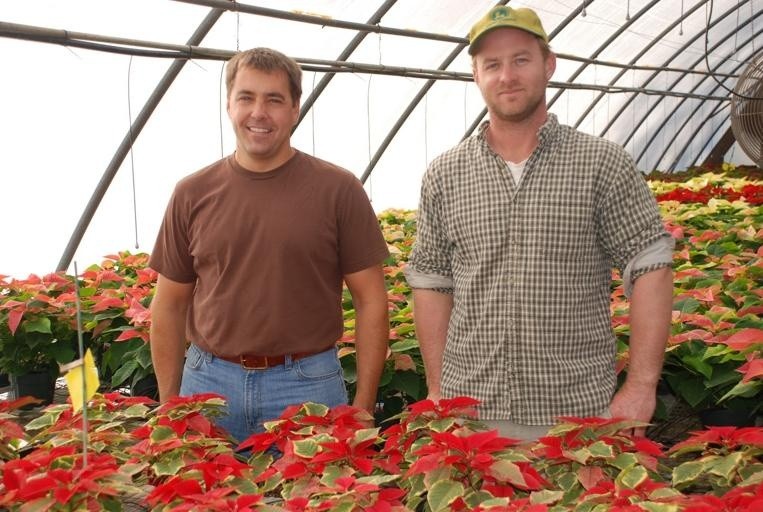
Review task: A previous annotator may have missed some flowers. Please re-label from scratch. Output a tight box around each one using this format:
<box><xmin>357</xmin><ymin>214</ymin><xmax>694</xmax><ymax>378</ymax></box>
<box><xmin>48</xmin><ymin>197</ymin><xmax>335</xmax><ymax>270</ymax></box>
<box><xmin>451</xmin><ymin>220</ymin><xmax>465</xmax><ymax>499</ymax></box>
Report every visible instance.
<box><xmin>0</xmin><ymin>255</ymin><xmax>93</xmax><ymax>370</ymax></box>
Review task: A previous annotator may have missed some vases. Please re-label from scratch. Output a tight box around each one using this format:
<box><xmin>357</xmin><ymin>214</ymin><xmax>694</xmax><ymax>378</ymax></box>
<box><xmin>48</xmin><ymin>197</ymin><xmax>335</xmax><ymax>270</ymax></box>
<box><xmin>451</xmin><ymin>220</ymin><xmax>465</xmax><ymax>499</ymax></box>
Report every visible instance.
<box><xmin>4</xmin><ymin>365</ymin><xmax>59</xmax><ymax>407</ymax></box>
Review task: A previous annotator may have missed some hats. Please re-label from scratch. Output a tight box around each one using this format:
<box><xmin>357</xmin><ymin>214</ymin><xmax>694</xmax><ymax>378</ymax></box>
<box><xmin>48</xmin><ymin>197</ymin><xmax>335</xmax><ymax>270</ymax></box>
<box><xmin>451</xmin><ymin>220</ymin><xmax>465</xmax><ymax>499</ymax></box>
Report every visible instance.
<box><xmin>467</xmin><ymin>6</ymin><xmax>549</xmax><ymax>54</ymax></box>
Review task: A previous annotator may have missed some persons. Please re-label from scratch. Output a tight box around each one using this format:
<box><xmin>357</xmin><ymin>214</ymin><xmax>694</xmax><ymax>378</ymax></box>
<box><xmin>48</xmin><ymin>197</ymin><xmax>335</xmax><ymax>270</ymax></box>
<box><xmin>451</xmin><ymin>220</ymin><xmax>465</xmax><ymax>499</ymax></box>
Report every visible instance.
<box><xmin>405</xmin><ymin>5</ymin><xmax>674</xmax><ymax>427</ymax></box>
<box><xmin>147</xmin><ymin>46</ymin><xmax>389</xmax><ymax>462</ymax></box>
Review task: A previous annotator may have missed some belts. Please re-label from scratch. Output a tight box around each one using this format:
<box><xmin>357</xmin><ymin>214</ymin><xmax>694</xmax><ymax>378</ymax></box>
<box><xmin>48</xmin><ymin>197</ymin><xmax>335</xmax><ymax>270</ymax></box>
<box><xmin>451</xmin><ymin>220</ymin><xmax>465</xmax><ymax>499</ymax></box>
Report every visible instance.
<box><xmin>219</xmin><ymin>352</ymin><xmax>309</xmax><ymax>370</ymax></box>
